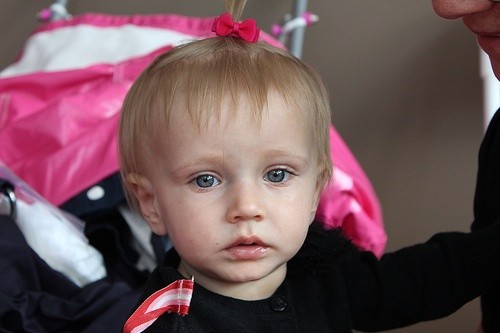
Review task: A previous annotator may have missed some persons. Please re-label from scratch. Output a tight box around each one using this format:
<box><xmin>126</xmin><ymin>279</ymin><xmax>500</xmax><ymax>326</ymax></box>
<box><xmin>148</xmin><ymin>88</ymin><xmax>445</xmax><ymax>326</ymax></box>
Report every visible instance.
<box><xmin>117</xmin><ymin>0</ymin><xmax>500</xmax><ymax>333</ymax></box>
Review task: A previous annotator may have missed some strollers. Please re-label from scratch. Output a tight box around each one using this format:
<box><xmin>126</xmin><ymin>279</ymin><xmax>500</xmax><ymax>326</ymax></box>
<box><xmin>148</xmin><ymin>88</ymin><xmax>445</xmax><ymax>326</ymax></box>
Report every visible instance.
<box><xmin>0</xmin><ymin>1</ymin><xmax>389</xmax><ymax>256</ymax></box>
